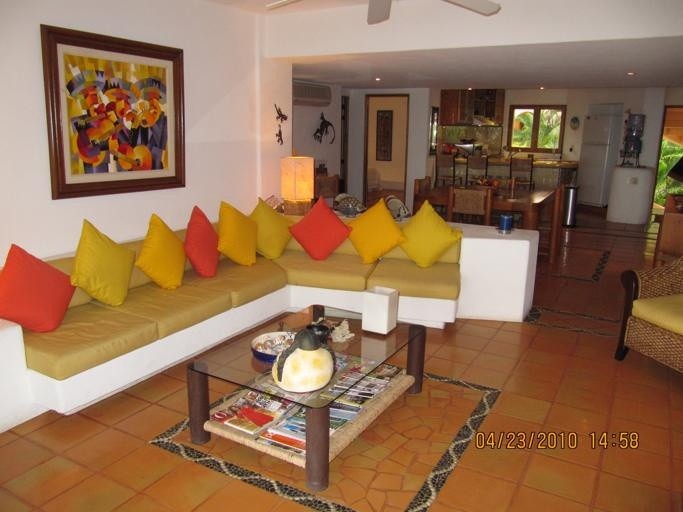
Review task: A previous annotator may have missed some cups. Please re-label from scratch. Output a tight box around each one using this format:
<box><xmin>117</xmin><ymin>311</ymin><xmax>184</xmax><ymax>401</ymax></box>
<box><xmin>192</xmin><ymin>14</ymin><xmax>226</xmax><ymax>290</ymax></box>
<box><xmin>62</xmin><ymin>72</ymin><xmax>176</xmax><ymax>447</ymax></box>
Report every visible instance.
<box><xmin>343</xmin><ymin>203</ymin><xmax>357</xmax><ymax>218</ymax></box>
<box><xmin>497</xmin><ymin>214</ymin><xmax>513</xmax><ymax>234</ymax></box>
<box><xmin>306</xmin><ymin>323</ymin><xmax>330</xmax><ymax>344</ymax></box>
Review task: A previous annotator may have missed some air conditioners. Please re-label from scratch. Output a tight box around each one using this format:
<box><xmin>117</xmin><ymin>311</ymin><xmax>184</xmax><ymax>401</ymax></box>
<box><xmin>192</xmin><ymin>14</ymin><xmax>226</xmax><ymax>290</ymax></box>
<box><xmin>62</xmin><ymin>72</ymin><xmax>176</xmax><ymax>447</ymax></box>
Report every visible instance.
<box><xmin>292</xmin><ymin>81</ymin><xmax>332</xmax><ymax>108</ymax></box>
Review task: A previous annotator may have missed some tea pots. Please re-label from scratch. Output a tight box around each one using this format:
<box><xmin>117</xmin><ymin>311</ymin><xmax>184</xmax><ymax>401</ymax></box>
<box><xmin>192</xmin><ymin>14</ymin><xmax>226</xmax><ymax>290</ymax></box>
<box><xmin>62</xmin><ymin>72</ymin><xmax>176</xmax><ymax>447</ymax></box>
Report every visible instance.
<box><xmin>271</xmin><ymin>330</ymin><xmax>335</xmax><ymax>393</ymax></box>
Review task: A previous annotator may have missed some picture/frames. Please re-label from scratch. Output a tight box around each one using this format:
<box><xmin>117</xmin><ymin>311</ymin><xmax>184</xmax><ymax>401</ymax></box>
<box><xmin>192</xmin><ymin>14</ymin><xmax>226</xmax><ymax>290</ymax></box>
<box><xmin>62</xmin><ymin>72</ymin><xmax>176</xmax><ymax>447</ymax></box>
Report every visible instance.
<box><xmin>39</xmin><ymin>21</ymin><xmax>187</xmax><ymax>201</ymax></box>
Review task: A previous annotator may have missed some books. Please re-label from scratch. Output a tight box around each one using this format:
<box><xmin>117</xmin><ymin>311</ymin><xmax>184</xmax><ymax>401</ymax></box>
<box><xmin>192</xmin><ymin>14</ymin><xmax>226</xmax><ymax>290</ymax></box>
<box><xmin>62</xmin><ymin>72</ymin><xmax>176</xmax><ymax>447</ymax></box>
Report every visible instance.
<box><xmin>209</xmin><ymin>349</ymin><xmax>409</xmax><ymax>461</ymax></box>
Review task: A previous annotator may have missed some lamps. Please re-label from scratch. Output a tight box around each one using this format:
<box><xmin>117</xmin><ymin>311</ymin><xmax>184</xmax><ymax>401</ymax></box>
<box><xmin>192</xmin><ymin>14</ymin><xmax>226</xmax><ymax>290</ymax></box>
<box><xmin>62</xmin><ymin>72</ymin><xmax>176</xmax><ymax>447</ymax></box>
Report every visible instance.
<box><xmin>280</xmin><ymin>155</ymin><xmax>316</xmax><ymax>213</ymax></box>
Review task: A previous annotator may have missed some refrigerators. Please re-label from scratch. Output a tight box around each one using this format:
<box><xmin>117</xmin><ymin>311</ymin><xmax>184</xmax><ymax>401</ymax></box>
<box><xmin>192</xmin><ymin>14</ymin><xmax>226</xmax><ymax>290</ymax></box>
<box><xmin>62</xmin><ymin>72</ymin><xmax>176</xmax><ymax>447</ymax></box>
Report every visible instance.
<box><xmin>576</xmin><ymin>102</ymin><xmax>623</xmax><ymax>209</ymax></box>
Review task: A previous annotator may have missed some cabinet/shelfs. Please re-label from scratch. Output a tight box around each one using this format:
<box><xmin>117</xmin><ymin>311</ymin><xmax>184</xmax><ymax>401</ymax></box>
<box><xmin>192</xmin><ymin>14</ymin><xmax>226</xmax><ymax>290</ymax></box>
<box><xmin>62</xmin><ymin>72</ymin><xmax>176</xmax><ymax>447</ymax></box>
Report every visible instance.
<box><xmin>439</xmin><ymin>89</ymin><xmax>459</xmax><ymax>124</ymax></box>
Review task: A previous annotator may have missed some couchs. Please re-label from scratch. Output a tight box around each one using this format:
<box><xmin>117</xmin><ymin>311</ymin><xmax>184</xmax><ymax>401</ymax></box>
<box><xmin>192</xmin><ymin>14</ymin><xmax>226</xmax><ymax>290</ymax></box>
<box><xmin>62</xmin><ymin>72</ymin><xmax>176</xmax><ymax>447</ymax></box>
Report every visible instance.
<box><xmin>0</xmin><ymin>205</ymin><xmax>461</xmax><ymax>416</ymax></box>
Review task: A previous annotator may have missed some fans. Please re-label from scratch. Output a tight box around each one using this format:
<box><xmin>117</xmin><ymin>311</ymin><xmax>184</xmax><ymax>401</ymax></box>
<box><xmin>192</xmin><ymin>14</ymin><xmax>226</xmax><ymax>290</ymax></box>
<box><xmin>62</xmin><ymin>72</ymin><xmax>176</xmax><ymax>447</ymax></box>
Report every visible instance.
<box><xmin>268</xmin><ymin>0</ymin><xmax>501</xmax><ymax>27</ymax></box>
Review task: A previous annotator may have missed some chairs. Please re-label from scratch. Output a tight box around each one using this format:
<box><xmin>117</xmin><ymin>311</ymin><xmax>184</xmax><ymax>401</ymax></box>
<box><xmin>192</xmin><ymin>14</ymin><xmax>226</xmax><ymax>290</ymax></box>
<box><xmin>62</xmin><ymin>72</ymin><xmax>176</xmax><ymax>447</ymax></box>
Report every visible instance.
<box><xmin>616</xmin><ymin>253</ymin><xmax>683</xmax><ymax>374</ymax></box>
<box><xmin>413</xmin><ymin>151</ymin><xmax>566</xmax><ymax>264</ymax></box>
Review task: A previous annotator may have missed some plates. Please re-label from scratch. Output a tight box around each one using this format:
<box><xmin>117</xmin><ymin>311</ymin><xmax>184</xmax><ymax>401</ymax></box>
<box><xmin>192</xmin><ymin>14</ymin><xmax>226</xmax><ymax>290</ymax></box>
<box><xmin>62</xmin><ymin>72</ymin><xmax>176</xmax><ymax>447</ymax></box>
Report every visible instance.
<box><xmin>248</xmin><ymin>331</ymin><xmax>296</xmax><ymax>362</ymax></box>
